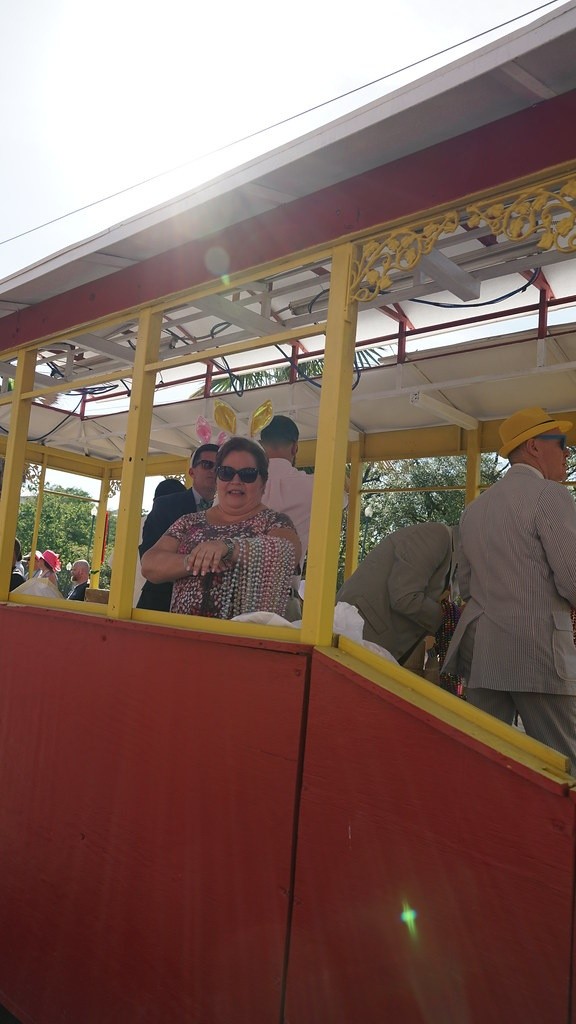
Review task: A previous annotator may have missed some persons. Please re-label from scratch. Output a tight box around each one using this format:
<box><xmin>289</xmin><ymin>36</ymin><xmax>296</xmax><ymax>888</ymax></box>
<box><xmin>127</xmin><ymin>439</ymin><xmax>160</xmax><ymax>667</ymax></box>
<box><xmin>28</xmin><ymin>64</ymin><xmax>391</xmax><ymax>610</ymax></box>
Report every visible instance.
<box><xmin>6</xmin><ymin>537</ymin><xmax>27</xmax><ymax>592</ymax></box>
<box><xmin>36</xmin><ymin>549</ymin><xmax>61</xmax><ymax>589</ymax></box>
<box><xmin>66</xmin><ymin>559</ymin><xmax>91</xmax><ymax>601</ymax></box>
<box><xmin>22</xmin><ymin>549</ymin><xmax>44</xmax><ymax>582</ymax></box>
<box><xmin>254</xmin><ymin>412</ymin><xmax>351</xmax><ymax>623</ymax></box>
<box><xmin>13</xmin><ymin>549</ymin><xmax>25</xmax><ymax>577</ymax></box>
<box><xmin>435</xmin><ymin>403</ymin><xmax>576</xmax><ymax>773</ymax></box>
<box><xmin>134</xmin><ymin>442</ymin><xmax>219</xmax><ymax>614</ymax></box>
<box><xmin>333</xmin><ymin>522</ymin><xmax>462</xmax><ymax>678</ymax></box>
<box><xmin>139</xmin><ymin>437</ymin><xmax>304</xmax><ymax>625</ymax></box>
<box><xmin>105</xmin><ymin>476</ymin><xmax>188</xmax><ymax>610</ymax></box>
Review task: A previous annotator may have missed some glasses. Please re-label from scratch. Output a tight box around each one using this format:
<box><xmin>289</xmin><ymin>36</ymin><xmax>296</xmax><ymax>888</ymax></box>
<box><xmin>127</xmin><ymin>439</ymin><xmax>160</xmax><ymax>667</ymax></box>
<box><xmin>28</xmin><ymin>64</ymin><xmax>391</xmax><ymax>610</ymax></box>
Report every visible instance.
<box><xmin>216</xmin><ymin>465</ymin><xmax>260</xmax><ymax>484</ymax></box>
<box><xmin>194</xmin><ymin>460</ymin><xmax>216</xmax><ymax>471</ymax></box>
<box><xmin>533</xmin><ymin>434</ymin><xmax>567</xmax><ymax>450</ymax></box>
<box><xmin>28</xmin><ymin>559</ymin><xmax>30</xmax><ymax>561</ymax></box>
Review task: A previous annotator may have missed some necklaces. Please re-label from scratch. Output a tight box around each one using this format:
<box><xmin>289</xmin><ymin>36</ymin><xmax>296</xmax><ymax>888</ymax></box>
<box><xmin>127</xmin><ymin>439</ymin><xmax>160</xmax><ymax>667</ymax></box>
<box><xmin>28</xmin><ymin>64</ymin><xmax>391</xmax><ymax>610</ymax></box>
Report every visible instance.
<box><xmin>437</xmin><ymin>595</ymin><xmax>468</xmax><ymax>703</ymax></box>
<box><xmin>568</xmin><ymin>603</ymin><xmax>576</xmax><ymax>647</ymax></box>
<box><xmin>212</xmin><ymin>532</ymin><xmax>298</xmax><ymax>626</ymax></box>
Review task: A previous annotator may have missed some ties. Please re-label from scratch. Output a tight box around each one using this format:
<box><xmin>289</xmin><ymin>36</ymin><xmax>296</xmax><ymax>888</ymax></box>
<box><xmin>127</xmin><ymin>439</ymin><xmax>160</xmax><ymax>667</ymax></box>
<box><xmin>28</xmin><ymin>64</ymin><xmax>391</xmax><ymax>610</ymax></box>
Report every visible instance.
<box><xmin>201</xmin><ymin>499</ymin><xmax>213</xmax><ymax>511</ymax></box>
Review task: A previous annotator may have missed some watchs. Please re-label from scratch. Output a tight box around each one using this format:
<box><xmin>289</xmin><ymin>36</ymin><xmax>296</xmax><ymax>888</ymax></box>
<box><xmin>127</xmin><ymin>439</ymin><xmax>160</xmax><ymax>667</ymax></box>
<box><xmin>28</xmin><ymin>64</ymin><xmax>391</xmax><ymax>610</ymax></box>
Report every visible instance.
<box><xmin>219</xmin><ymin>537</ymin><xmax>236</xmax><ymax>562</ymax></box>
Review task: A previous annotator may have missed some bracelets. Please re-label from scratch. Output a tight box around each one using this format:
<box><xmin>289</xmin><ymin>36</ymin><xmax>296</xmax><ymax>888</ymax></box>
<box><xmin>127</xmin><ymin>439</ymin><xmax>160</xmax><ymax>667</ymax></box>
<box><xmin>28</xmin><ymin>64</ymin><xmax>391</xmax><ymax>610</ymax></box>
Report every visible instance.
<box><xmin>183</xmin><ymin>551</ymin><xmax>196</xmax><ymax>576</ymax></box>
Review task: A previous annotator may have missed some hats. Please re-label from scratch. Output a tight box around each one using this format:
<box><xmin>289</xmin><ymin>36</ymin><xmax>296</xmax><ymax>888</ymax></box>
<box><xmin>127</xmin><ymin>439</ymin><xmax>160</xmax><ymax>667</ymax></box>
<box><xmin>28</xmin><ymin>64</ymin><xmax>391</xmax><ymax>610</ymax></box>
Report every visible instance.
<box><xmin>41</xmin><ymin>550</ymin><xmax>61</xmax><ymax>572</ymax></box>
<box><xmin>497</xmin><ymin>406</ymin><xmax>572</xmax><ymax>458</ymax></box>
<box><xmin>23</xmin><ymin>549</ymin><xmax>42</xmax><ymax>560</ymax></box>
